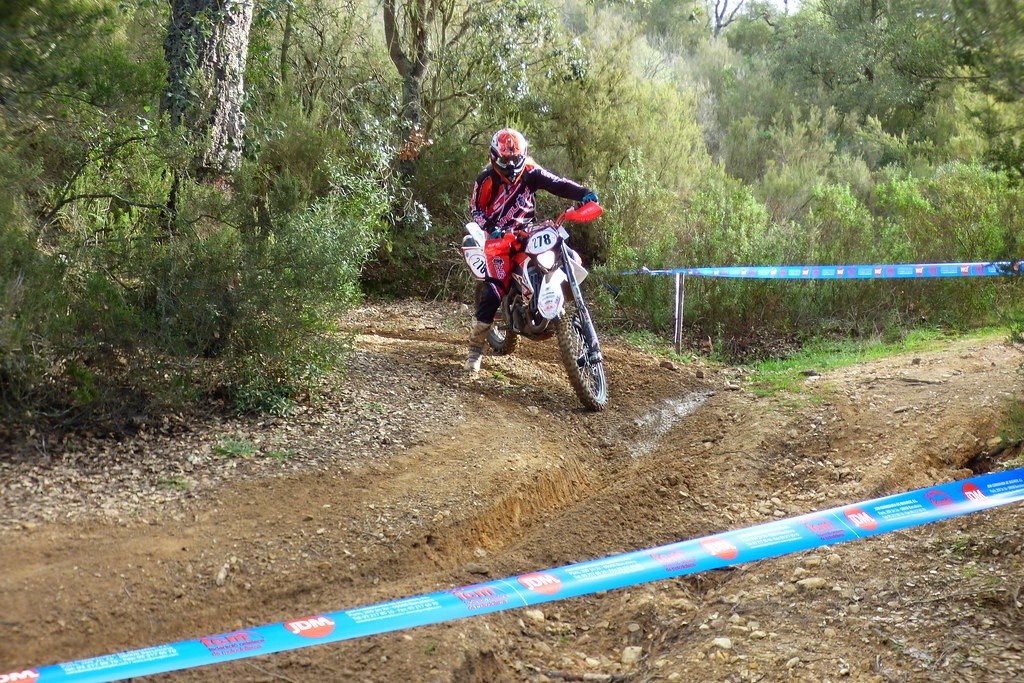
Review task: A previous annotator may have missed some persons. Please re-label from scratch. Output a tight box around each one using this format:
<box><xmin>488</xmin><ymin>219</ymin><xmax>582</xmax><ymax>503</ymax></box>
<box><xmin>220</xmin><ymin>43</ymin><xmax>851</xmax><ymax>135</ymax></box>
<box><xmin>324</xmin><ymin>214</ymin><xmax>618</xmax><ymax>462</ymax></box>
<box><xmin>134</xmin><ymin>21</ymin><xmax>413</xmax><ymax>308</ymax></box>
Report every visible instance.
<box><xmin>462</xmin><ymin>128</ymin><xmax>600</xmax><ymax>372</ymax></box>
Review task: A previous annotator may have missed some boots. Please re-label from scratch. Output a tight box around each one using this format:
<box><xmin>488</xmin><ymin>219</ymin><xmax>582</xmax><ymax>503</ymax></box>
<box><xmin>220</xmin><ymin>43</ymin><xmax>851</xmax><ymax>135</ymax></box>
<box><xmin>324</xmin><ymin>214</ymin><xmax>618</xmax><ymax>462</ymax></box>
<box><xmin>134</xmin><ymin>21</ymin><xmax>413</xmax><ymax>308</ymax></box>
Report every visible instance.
<box><xmin>464</xmin><ymin>322</ymin><xmax>491</xmax><ymax>372</ymax></box>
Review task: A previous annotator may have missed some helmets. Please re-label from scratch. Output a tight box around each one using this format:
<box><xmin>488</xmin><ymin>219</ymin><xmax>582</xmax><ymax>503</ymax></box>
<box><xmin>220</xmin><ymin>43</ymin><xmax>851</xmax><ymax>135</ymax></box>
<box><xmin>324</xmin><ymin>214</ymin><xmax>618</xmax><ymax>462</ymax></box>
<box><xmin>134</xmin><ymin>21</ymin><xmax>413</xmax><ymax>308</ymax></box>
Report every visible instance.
<box><xmin>490</xmin><ymin>129</ymin><xmax>527</xmax><ymax>187</ymax></box>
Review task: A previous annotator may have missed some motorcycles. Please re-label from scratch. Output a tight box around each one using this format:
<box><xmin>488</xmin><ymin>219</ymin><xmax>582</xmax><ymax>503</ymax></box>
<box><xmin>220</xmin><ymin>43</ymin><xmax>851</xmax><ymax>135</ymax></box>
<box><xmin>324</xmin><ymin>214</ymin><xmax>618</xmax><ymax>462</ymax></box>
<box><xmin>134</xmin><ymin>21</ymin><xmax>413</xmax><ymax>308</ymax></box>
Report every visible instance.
<box><xmin>458</xmin><ymin>204</ymin><xmax>612</xmax><ymax>411</ymax></box>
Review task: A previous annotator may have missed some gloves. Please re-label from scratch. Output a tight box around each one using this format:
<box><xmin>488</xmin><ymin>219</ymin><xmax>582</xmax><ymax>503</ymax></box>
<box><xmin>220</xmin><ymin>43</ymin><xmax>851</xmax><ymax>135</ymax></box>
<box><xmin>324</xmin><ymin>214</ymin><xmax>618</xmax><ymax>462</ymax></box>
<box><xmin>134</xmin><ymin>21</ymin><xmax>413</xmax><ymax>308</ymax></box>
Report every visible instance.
<box><xmin>583</xmin><ymin>193</ymin><xmax>598</xmax><ymax>204</ymax></box>
<box><xmin>488</xmin><ymin>229</ymin><xmax>501</xmax><ymax>240</ymax></box>
